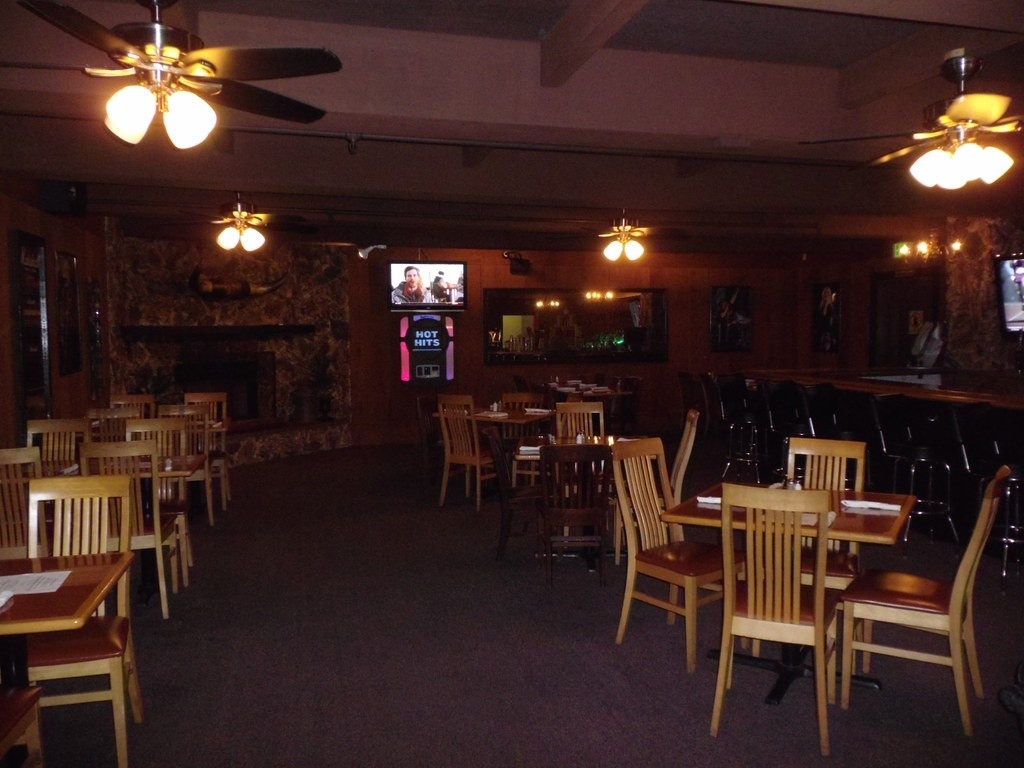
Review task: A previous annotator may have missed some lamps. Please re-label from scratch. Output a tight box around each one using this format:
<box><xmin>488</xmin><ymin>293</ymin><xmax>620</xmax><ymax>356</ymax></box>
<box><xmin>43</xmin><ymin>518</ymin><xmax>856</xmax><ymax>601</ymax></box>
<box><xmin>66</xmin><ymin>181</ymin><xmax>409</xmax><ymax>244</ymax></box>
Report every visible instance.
<box><xmin>216</xmin><ymin>222</ymin><xmax>266</xmax><ymax>251</ymax></box>
<box><xmin>603</xmin><ymin>234</ymin><xmax>644</xmax><ymax>262</ymax></box>
<box><xmin>503</xmin><ymin>251</ymin><xmax>534</xmax><ymax>276</ymax></box>
<box><xmin>909</xmin><ymin>142</ymin><xmax>1014</xmax><ymax>190</ymax></box>
<box><xmin>104</xmin><ymin>77</ymin><xmax>218</xmax><ymax>149</ymax></box>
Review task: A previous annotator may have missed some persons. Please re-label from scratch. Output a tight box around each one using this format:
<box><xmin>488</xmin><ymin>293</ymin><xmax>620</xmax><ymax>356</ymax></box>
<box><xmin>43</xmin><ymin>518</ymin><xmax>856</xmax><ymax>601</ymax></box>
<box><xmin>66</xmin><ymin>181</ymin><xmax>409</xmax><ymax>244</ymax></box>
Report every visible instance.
<box><xmin>391</xmin><ymin>266</ymin><xmax>464</xmax><ymax>303</ymax></box>
<box><xmin>718</xmin><ymin>288</ymin><xmax>743</xmax><ymax>335</ymax></box>
<box><xmin>815</xmin><ymin>287</ymin><xmax>839</xmax><ymax>352</ymax></box>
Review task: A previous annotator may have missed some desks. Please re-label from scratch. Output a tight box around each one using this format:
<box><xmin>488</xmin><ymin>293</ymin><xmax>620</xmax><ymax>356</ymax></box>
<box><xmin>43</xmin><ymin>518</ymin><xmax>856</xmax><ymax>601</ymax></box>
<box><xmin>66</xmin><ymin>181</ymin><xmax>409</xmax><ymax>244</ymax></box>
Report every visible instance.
<box><xmin>540</xmin><ymin>382</ymin><xmax>634</xmax><ymax>434</ymax></box>
<box><xmin>0</xmin><ymin>551</ymin><xmax>136</xmax><ymax>768</ymax></box>
<box><xmin>514</xmin><ymin>436</ymin><xmax>656</xmax><ymax>572</ymax></box>
<box><xmin>75</xmin><ymin>416</ymin><xmax>231</xmax><ymax>521</ymax></box>
<box><xmin>432</xmin><ymin>407</ymin><xmax>556</xmax><ymax>502</ymax></box>
<box><xmin>0</xmin><ymin>454</ymin><xmax>208</xmax><ymax>608</ymax></box>
<box><xmin>723</xmin><ymin>370</ymin><xmax>1024</xmax><ymax>566</ymax></box>
<box><xmin>660</xmin><ymin>481</ymin><xmax>918</xmax><ymax>706</ymax></box>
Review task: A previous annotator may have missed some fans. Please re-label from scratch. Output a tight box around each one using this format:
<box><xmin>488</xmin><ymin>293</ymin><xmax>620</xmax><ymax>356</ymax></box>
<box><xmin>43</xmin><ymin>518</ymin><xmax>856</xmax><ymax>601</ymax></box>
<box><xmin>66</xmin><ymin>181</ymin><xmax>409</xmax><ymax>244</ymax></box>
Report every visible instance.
<box><xmin>545</xmin><ymin>208</ymin><xmax>688</xmax><ymax>243</ymax></box>
<box><xmin>0</xmin><ymin>0</ymin><xmax>343</xmax><ymax>125</ymax></box>
<box><xmin>148</xmin><ymin>193</ymin><xmax>320</xmax><ymax>234</ymax></box>
<box><xmin>797</xmin><ymin>46</ymin><xmax>1024</xmax><ymax>173</ymax></box>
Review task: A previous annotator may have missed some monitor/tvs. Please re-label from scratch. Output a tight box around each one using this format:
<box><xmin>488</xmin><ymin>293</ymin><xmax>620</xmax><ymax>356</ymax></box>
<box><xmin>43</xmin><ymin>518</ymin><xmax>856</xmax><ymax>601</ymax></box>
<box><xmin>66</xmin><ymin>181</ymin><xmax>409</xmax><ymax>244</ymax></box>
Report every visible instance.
<box><xmin>994</xmin><ymin>252</ymin><xmax>1024</xmax><ymax>337</ymax></box>
<box><xmin>388</xmin><ymin>260</ymin><xmax>467</xmax><ymax>312</ymax></box>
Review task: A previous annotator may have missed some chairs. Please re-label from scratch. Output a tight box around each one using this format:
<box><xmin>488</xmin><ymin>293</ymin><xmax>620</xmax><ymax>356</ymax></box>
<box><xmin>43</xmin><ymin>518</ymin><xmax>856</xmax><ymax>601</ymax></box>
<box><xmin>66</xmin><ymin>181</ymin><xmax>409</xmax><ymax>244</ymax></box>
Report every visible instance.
<box><xmin>0</xmin><ymin>392</ymin><xmax>231</xmax><ymax>768</ymax></box>
<box><xmin>676</xmin><ymin>370</ymin><xmax>1024</xmax><ymax>596</ymax></box>
<box><xmin>612</xmin><ymin>437</ymin><xmax>751</xmax><ymax>674</ymax></box>
<box><xmin>709</xmin><ymin>481</ymin><xmax>842</xmax><ymax>756</ymax></box>
<box><xmin>752</xmin><ymin>436</ymin><xmax>868</xmax><ymax>675</ymax></box>
<box><xmin>415</xmin><ymin>372</ymin><xmax>700</xmax><ymax>588</ymax></box>
<box><xmin>841</xmin><ymin>465</ymin><xmax>1012</xmax><ymax>737</ymax></box>
